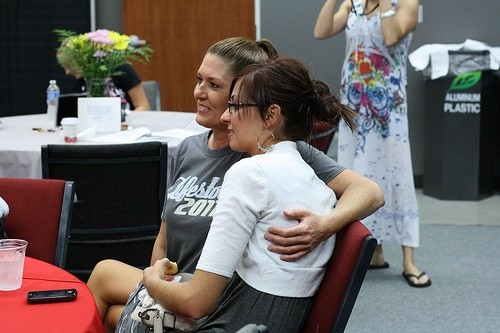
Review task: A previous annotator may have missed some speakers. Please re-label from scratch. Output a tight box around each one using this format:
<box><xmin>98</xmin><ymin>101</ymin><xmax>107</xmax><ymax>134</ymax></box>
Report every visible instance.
<box><xmin>421</xmin><ymin>69</ymin><xmax>500</xmax><ymax>201</ymax></box>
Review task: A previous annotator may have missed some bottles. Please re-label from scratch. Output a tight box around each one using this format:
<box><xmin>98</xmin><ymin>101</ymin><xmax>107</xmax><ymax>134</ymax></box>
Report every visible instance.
<box><xmin>45</xmin><ymin>79</ymin><xmax>60</xmax><ymax>128</ymax></box>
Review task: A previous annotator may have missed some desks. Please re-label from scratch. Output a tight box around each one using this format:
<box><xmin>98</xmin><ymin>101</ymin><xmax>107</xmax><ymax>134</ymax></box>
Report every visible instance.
<box><xmin>0</xmin><ymin>252</ymin><xmax>110</xmax><ymax>333</ymax></box>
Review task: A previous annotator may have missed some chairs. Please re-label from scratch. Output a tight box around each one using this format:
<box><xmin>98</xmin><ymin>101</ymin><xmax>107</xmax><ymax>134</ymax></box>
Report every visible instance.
<box><xmin>0</xmin><ymin>177</ymin><xmax>76</xmax><ymax>268</ymax></box>
<box><xmin>302</xmin><ymin>217</ymin><xmax>379</xmax><ymax>333</ymax></box>
<box><xmin>40</xmin><ymin>140</ymin><xmax>170</xmax><ymax>269</ymax></box>
<box><xmin>142</xmin><ymin>80</ymin><xmax>163</xmax><ymax>111</ymax></box>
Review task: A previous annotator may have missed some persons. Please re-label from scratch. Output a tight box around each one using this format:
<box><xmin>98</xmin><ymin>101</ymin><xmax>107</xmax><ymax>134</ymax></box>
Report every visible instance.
<box><xmin>313</xmin><ymin>0</ymin><xmax>432</xmax><ymax>287</ymax></box>
<box><xmin>57</xmin><ymin>36</ymin><xmax>151</xmax><ymax>113</ymax></box>
<box><xmin>143</xmin><ymin>57</ymin><xmax>338</xmax><ymax>333</ymax></box>
<box><xmin>88</xmin><ymin>37</ymin><xmax>386</xmax><ymax>333</ymax></box>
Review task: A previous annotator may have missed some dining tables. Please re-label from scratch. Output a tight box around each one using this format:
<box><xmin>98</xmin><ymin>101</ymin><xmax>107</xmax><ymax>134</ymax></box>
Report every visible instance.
<box><xmin>0</xmin><ymin>108</ymin><xmax>212</xmax><ymax>180</ymax></box>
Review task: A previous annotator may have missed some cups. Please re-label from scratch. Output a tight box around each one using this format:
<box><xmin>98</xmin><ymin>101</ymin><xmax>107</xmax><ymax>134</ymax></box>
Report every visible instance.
<box><xmin>0</xmin><ymin>238</ymin><xmax>28</xmax><ymax>291</ymax></box>
<box><xmin>60</xmin><ymin>117</ymin><xmax>79</xmax><ymax>143</ymax></box>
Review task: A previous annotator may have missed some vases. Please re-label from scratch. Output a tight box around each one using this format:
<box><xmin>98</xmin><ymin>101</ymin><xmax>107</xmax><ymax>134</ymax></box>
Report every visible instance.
<box><xmin>85</xmin><ymin>81</ymin><xmax>108</xmax><ymax>97</ymax></box>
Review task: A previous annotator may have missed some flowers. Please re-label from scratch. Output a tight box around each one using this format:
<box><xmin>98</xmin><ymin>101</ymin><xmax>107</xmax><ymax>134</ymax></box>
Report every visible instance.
<box><xmin>51</xmin><ymin>23</ymin><xmax>157</xmax><ymax>84</ymax></box>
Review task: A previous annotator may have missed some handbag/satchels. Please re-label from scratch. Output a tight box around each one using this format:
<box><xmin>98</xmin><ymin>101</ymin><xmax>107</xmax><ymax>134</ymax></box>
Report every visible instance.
<box><xmin>115</xmin><ymin>272</ymin><xmax>212</xmax><ymax>333</ymax></box>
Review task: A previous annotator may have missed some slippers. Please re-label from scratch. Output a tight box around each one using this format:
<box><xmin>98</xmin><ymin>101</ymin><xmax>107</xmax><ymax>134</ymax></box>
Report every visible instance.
<box><xmin>367</xmin><ymin>261</ymin><xmax>389</xmax><ymax>269</ymax></box>
<box><xmin>402</xmin><ymin>270</ymin><xmax>431</xmax><ymax>287</ymax></box>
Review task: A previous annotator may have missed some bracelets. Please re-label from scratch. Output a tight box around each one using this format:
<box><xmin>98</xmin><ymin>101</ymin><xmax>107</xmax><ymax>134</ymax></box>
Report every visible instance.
<box><xmin>380</xmin><ymin>10</ymin><xmax>395</xmax><ymax>18</ymax></box>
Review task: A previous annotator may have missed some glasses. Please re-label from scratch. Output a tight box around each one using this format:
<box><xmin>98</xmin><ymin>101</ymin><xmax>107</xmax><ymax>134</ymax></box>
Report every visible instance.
<box><xmin>228</xmin><ymin>101</ymin><xmax>269</xmax><ymax>113</ymax></box>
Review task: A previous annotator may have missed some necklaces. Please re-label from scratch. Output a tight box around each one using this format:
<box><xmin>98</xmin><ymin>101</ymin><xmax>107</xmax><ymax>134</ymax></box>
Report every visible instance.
<box><xmin>362</xmin><ymin>0</ymin><xmax>379</xmax><ymax>15</ymax></box>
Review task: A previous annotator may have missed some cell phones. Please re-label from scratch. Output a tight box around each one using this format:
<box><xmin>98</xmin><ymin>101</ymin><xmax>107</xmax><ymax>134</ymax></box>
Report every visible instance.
<box><xmin>27</xmin><ymin>289</ymin><xmax>77</xmax><ymax>303</ymax></box>
